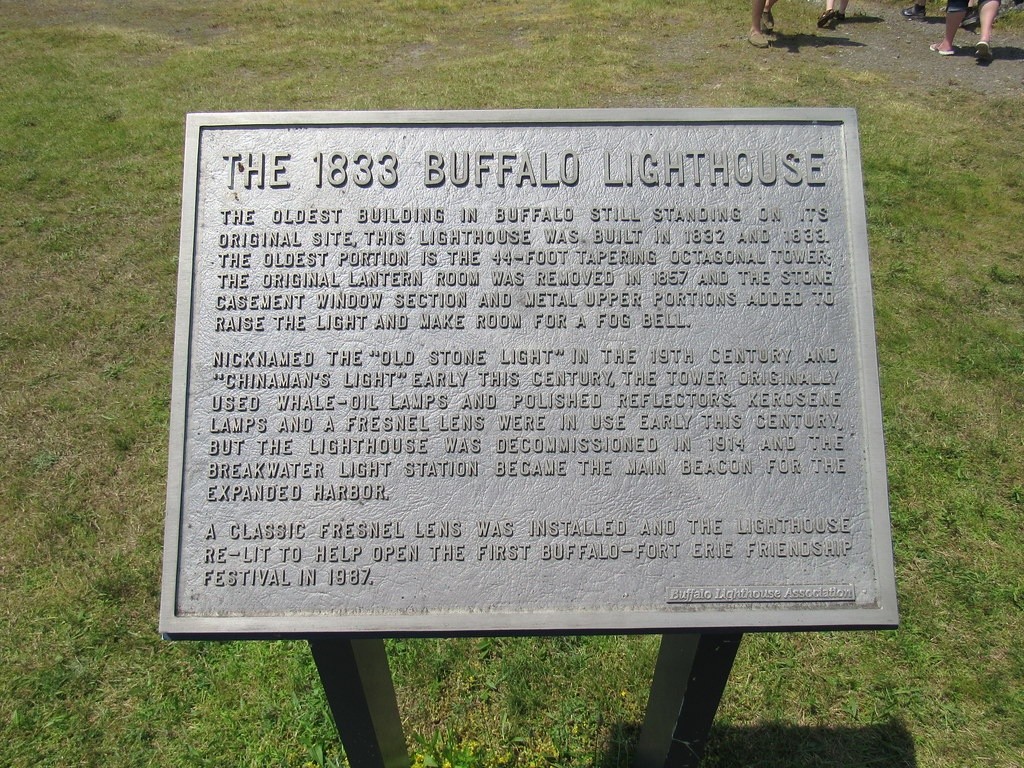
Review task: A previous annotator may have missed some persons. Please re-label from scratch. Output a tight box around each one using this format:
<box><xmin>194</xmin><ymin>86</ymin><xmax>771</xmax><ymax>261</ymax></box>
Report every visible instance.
<box><xmin>817</xmin><ymin>0</ymin><xmax>849</xmax><ymax>26</ymax></box>
<box><xmin>747</xmin><ymin>0</ymin><xmax>778</xmax><ymax>47</ymax></box>
<box><xmin>929</xmin><ymin>0</ymin><xmax>1001</xmax><ymax>58</ymax></box>
<box><xmin>901</xmin><ymin>0</ymin><xmax>975</xmax><ymax>22</ymax></box>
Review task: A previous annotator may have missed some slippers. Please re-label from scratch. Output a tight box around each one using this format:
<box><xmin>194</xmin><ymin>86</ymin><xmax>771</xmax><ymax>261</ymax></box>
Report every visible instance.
<box><xmin>930</xmin><ymin>41</ymin><xmax>955</xmax><ymax>55</ymax></box>
<box><xmin>975</xmin><ymin>41</ymin><xmax>992</xmax><ymax>60</ymax></box>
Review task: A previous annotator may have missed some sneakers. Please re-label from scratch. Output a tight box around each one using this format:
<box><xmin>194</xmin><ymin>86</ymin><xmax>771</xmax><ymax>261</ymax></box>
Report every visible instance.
<box><xmin>763</xmin><ymin>11</ymin><xmax>775</xmax><ymax>30</ymax></box>
<box><xmin>816</xmin><ymin>9</ymin><xmax>834</xmax><ymax>27</ymax></box>
<box><xmin>748</xmin><ymin>30</ymin><xmax>769</xmax><ymax>48</ymax></box>
<box><xmin>831</xmin><ymin>10</ymin><xmax>845</xmax><ymax>19</ymax></box>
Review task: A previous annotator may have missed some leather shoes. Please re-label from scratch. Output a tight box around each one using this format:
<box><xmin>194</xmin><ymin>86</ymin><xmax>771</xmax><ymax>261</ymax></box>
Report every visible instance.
<box><xmin>959</xmin><ymin>8</ymin><xmax>979</xmax><ymax>28</ymax></box>
<box><xmin>899</xmin><ymin>4</ymin><xmax>926</xmax><ymax>19</ymax></box>
